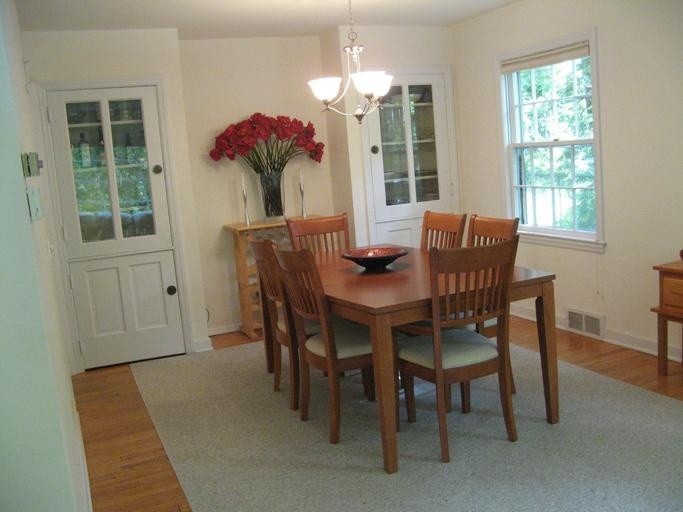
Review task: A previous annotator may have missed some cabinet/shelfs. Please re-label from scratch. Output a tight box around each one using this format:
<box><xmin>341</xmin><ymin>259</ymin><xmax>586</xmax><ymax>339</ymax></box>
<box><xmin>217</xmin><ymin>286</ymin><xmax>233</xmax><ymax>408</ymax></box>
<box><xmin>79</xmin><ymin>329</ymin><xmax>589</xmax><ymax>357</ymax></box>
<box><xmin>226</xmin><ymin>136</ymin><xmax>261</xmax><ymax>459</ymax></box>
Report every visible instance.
<box><xmin>365</xmin><ymin>71</ymin><xmax>462</xmax><ymax>251</ymax></box>
<box><xmin>45</xmin><ymin>85</ymin><xmax>186</xmax><ymax>370</ymax></box>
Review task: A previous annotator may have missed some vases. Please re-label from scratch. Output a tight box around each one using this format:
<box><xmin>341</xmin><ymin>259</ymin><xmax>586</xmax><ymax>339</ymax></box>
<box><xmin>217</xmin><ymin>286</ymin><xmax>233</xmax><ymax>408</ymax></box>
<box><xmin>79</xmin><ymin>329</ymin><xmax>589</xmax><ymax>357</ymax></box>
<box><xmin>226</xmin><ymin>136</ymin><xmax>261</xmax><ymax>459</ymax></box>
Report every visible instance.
<box><xmin>257</xmin><ymin>169</ymin><xmax>285</xmax><ymax>224</ymax></box>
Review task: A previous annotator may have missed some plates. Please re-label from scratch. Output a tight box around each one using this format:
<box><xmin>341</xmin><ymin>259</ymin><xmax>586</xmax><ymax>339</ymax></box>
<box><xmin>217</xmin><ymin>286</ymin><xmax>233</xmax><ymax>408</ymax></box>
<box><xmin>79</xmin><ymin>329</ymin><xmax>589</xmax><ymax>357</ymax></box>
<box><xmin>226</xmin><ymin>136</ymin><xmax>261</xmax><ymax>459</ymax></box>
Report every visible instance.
<box><xmin>406</xmin><ymin>169</ymin><xmax>435</xmax><ymax>175</ymax></box>
<box><xmin>384</xmin><ymin>170</ymin><xmax>403</xmax><ymax>177</ymax></box>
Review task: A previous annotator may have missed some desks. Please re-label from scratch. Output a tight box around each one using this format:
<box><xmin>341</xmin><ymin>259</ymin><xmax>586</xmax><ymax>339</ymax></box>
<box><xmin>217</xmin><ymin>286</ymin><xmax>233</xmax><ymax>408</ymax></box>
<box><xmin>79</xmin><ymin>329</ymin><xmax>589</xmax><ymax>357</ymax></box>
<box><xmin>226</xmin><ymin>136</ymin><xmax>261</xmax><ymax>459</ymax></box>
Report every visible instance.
<box><xmin>650</xmin><ymin>258</ymin><xmax>683</xmax><ymax>377</ymax></box>
<box><xmin>223</xmin><ymin>214</ymin><xmax>321</xmax><ymax>342</ymax></box>
<box><xmin>259</xmin><ymin>245</ymin><xmax>559</xmax><ymax>474</ymax></box>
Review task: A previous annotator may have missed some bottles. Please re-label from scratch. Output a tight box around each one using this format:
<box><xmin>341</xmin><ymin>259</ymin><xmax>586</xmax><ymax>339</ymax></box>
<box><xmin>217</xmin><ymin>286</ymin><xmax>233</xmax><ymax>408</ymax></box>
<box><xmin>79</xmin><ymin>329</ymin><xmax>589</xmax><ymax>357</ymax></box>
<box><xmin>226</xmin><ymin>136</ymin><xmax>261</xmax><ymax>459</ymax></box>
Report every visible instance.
<box><xmin>66</xmin><ymin>126</ymin><xmax>149</xmax><ymax>166</ymax></box>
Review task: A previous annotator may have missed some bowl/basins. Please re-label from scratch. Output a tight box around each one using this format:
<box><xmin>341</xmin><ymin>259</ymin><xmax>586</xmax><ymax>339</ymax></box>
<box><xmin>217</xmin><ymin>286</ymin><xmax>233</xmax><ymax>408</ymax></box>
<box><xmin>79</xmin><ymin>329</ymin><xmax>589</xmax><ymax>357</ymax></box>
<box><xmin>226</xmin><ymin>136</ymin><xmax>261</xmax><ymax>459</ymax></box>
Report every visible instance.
<box><xmin>341</xmin><ymin>248</ymin><xmax>409</xmax><ymax>272</ymax></box>
<box><xmin>390</xmin><ymin>93</ymin><xmax>424</xmax><ymax>103</ymax></box>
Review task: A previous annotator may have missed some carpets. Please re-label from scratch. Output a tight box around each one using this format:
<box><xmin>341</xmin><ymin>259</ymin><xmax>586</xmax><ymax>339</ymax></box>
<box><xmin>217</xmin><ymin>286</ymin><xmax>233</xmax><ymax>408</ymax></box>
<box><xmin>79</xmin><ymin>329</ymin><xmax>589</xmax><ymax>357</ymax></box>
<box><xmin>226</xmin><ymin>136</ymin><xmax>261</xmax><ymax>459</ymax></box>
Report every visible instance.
<box><xmin>128</xmin><ymin>336</ymin><xmax>683</xmax><ymax>512</ymax></box>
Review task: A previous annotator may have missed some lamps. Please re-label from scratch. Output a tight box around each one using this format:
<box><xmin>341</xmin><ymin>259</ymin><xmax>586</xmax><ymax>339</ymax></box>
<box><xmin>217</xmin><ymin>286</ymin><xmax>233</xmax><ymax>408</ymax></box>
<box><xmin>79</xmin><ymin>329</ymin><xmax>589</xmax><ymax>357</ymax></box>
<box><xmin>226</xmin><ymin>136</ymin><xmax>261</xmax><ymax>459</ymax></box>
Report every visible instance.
<box><xmin>307</xmin><ymin>0</ymin><xmax>394</xmax><ymax>125</ymax></box>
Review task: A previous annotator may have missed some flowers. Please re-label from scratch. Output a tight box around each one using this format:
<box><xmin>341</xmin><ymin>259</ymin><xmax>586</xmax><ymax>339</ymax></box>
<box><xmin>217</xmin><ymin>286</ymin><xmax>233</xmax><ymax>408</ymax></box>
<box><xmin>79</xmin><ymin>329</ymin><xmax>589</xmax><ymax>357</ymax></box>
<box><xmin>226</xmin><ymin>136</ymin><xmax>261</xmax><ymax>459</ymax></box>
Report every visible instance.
<box><xmin>210</xmin><ymin>112</ymin><xmax>325</xmax><ymax>218</ymax></box>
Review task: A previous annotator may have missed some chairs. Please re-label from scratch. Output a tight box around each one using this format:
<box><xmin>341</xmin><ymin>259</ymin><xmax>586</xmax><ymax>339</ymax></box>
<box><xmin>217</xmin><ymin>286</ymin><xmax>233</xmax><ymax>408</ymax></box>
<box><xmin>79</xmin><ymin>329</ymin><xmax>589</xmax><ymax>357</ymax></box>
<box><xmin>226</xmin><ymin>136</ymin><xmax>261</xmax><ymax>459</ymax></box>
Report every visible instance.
<box><xmin>250</xmin><ymin>239</ymin><xmax>368</xmax><ymax>412</ymax></box>
<box><xmin>396</xmin><ymin>234</ymin><xmax>519</xmax><ymax>462</ymax></box>
<box><xmin>421</xmin><ymin>211</ymin><xmax>467</xmax><ymax>252</ymax></box>
<box><xmin>285</xmin><ymin>213</ymin><xmax>350</xmax><ymax>254</ymax></box>
<box><xmin>437</xmin><ymin>214</ymin><xmax>520</xmax><ymax>414</ymax></box>
<box><xmin>272</xmin><ymin>244</ymin><xmax>418</xmax><ymax>443</ymax></box>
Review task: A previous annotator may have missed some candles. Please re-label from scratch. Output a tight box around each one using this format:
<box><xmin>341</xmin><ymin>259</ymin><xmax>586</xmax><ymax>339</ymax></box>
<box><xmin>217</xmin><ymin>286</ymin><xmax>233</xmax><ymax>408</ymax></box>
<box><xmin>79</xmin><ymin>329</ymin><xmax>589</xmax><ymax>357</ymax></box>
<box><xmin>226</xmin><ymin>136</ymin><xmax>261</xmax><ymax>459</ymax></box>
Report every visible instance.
<box><xmin>299</xmin><ymin>168</ymin><xmax>303</xmax><ymax>185</ymax></box>
<box><xmin>240</xmin><ymin>172</ymin><xmax>246</xmax><ymax>190</ymax></box>
